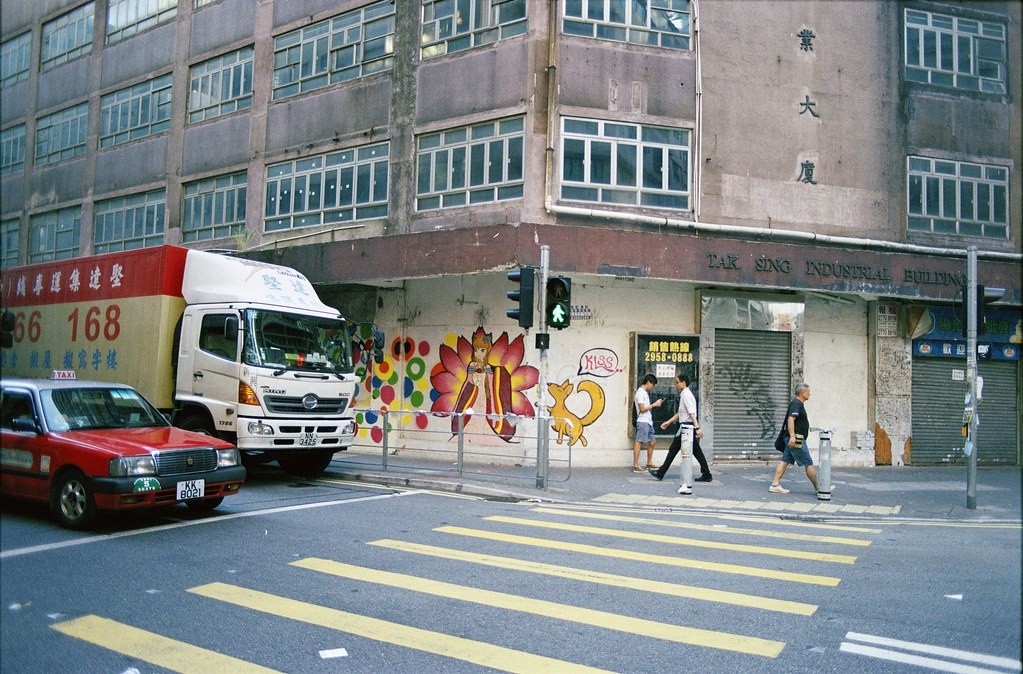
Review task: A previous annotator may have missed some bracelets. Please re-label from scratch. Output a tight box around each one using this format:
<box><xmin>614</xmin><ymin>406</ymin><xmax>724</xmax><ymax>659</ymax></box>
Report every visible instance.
<box><xmin>695</xmin><ymin>427</ymin><xmax>700</xmax><ymax>430</ymax></box>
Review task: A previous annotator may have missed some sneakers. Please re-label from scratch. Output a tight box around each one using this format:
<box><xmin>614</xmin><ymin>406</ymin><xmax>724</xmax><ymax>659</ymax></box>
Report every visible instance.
<box><xmin>815</xmin><ymin>485</ymin><xmax>835</xmax><ymax>495</ymax></box>
<box><xmin>768</xmin><ymin>484</ymin><xmax>790</xmax><ymax>493</ymax></box>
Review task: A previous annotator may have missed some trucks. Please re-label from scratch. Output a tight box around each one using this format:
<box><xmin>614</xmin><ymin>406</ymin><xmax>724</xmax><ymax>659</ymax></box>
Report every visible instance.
<box><xmin>0</xmin><ymin>244</ymin><xmax>384</xmax><ymax>480</ymax></box>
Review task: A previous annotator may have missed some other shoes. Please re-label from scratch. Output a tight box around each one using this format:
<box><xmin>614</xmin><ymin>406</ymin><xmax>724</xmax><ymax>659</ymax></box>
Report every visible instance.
<box><xmin>646</xmin><ymin>464</ymin><xmax>659</xmax><ymax>470</ymax></box>
<box><xmin>633</xmin><ymin>466</ymin><xmax>648</xmax><ymax>473</ymax></box>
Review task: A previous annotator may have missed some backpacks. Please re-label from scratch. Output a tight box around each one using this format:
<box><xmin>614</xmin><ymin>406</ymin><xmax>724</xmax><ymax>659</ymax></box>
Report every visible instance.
<box><xmin>632</xmin><ymin>390</ymin><xmax>645</xmax><ymax>427</ymax></box>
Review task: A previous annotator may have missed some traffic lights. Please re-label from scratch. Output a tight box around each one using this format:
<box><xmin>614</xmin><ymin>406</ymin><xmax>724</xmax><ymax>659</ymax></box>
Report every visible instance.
<box><xmin>506</xmin><ymin>267</ymin><xmax>534</xmax><ymax>329</ymax></box>
<box><xmin>546</xmin><ymin>275</ymin><xmax>572</xmax><ymax>331</ymax></box>
<box><xmin>977</xmin><ymin>284</ymin><xmax>1005</xmax><ymax>335</ymax></box>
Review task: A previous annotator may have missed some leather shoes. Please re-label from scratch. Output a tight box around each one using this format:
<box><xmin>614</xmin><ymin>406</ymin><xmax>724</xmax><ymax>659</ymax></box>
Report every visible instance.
<box><xmin>649</xmin><ymin>470</ymin><xmax>662</xmax><ymax>480</ymax></box>
<box><xmin>695</xmin><ymin>476</ymin><xmax>712</xmax><ymax>481</ymax></box>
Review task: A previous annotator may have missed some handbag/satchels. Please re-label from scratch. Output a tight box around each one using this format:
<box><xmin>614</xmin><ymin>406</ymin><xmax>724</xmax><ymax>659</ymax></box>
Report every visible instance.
<box><xmin>774</xmin><ymin>429</ymin><xmax>787</xmax><ymax>453</ymax></box>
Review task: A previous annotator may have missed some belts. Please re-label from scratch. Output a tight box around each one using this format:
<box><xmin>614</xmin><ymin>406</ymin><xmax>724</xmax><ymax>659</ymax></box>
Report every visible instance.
<box><xmin>680</xmin><ymin>422</ymin><xmax>694</xmax><ymax>425</ymax></box>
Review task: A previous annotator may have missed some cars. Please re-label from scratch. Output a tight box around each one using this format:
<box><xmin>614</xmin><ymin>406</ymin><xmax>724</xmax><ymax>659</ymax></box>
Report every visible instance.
<box><xmin>0</xmin><ymin>370</ymin><xmax>246</xmax><ymax>529</ymax></box>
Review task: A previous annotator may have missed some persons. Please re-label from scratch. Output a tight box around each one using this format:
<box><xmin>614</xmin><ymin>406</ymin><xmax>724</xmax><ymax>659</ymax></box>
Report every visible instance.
<box><xmin>769</xmin><ymin>383</ymin><xmax>836</xmax><ymax>494</ymax></box>
<box><xmin>650</xmin><ymin>374</ymin><xmax>712</xmax><ymax>481</ymax></box>
<box><xmin>633</xmin><ymin>374</ymin><xmax>664</xmax><ymax>473</ymax></box>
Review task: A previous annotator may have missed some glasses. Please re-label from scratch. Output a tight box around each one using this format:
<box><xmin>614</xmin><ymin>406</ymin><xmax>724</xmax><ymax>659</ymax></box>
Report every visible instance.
<box><xmin>650</xmin><ymin>381</ymin><xmax>655</xmax><ymax>387</ymax></box>
<box><xmin>676</xmin><ymin>380</ymin><xmax>682</xmax><ymax>385</ymax></box>
